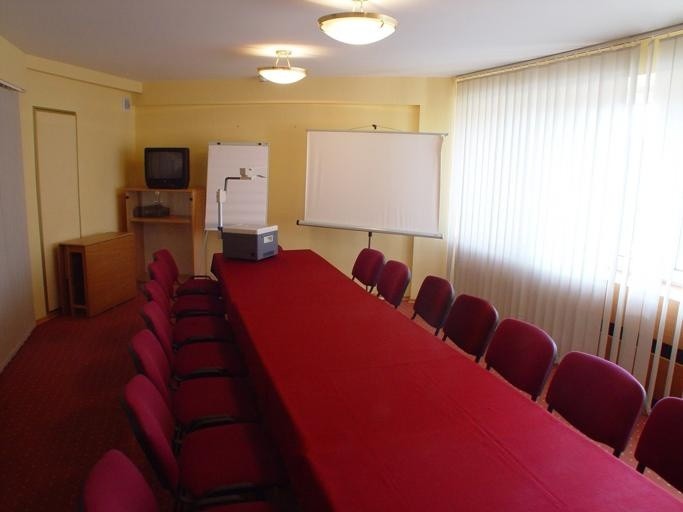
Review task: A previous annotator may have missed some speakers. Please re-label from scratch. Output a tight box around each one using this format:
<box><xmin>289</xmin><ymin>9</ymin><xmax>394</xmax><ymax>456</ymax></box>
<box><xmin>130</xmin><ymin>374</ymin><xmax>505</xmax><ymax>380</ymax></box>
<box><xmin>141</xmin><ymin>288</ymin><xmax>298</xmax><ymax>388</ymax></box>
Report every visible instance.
<box><xmin>133</xmin><ymin>205</ymin><xmax>170</xmax><ymax>217</ymax></box>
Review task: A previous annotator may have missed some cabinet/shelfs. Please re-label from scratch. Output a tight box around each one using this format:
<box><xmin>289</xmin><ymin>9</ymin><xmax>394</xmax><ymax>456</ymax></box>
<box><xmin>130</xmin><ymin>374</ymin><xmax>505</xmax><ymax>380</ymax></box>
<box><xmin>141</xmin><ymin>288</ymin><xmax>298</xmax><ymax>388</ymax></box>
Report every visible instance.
<box><xmin>120</xmin><ymin>185</ymin><xmax>208</xmax><ymax>279</ymax></box>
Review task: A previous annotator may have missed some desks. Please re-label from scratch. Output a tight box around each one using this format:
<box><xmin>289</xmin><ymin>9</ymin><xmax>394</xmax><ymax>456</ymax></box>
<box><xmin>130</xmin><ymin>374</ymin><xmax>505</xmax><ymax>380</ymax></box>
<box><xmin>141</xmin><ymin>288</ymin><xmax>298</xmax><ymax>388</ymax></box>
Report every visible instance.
<box><xmin>55</xmin><ymin>232</ymin><xmax>140</xmax><ymax>317</ymax></box>
<box><xmin>210</xmin><ymin>249</ymin><xmax>683</xmax><ymax>512</ymax></box>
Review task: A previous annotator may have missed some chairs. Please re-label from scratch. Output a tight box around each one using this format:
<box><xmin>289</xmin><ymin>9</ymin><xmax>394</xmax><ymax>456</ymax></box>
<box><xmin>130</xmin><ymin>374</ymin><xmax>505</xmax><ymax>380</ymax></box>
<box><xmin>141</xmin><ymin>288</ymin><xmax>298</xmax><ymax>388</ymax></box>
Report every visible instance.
<box><xmin>543</xmin><ymin>349</ymin><xmax>648</xmax><ymax>460</ymax></box>
<box><xmin>152</xmin><ymin>249</ymin><xmax>211</xmax><ymax>280</ymax></box>
<box><xmin>372</xmin><ymin>258</ymin><xmax>411</xmax><ymax>311</ymax></box>
<box><xmin>140</xmin><ymin>298</ymin><xmax>246</xmax><ymax>377</ymax></box>
<box><xmin>410</xmin><ymin>274</ymin><xmax>456</xmax><ymax>336</ymax></box>
<box><xmin>632</xmin><ymin>396</ymin><xmax>683</xmax><ymax>494</ymax></box>
<box><xmin>483</xmin><ymin>317</ymin><xmax>560</xmax><ymax>402</ymax></box>
<box><xmin>350</xmin><ymin>248</ymin><xmax>386</xmax><ymax>294</ymax></box>
<box><xmin>78</xmin><ymin>447</ymin><xmax>279</xmax><ymax>512</ymax></box>
<box><xmin>137</xmin><ymin>277</ymin><xmax>234</xmax><ymax>345</ymax></box>
<box><xmin>118</xmin><ymin>373</ymin><xmax>272</xmax><ymax>504</ymax></box>
<box><xmin>127</xmin><ymin>326</ymin><xmax>258</xmax><ymax>429</ymax></box>
<box><xmin>439</xmin><ymin>294</ymin><xmax>500</xmax><ymax>361</ymax></box>
<box><xmin>148</xmin><ymin>261</ymin><xmax>226</xmax><ymax>320</ymax></box>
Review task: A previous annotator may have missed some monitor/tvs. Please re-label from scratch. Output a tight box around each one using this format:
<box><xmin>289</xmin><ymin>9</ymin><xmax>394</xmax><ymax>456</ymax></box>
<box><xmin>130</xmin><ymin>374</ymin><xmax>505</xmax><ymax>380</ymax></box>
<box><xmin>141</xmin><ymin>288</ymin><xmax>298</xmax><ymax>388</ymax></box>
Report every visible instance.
<box><xmin>144</xmin><ymin>147</ymin><xmax>190</xmax><ymax>189</ymax></box>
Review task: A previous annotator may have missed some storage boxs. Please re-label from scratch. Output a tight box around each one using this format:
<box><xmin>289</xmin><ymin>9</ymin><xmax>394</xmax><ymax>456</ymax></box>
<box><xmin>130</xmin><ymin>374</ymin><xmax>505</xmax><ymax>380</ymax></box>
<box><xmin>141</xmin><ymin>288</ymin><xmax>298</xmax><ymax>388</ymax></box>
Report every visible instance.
<box><xmin>221</xmin><ymin>223</ymin><xmax>279</xmax><ymax>263</ymax></box>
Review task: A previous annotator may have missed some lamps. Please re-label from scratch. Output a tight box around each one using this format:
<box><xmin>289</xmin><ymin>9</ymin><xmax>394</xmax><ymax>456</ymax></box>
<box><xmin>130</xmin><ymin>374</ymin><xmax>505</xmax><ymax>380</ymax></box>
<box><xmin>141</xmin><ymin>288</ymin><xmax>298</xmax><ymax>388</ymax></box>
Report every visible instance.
<box><xmin>316</xmin><ymin>0</ymin><xmax>401</xmax><ymax>48</ymax></box>
<box><xmin>258</xmin><ymin>47</ymin><xmax>307</xmax><ymax>87</ymax></box>
